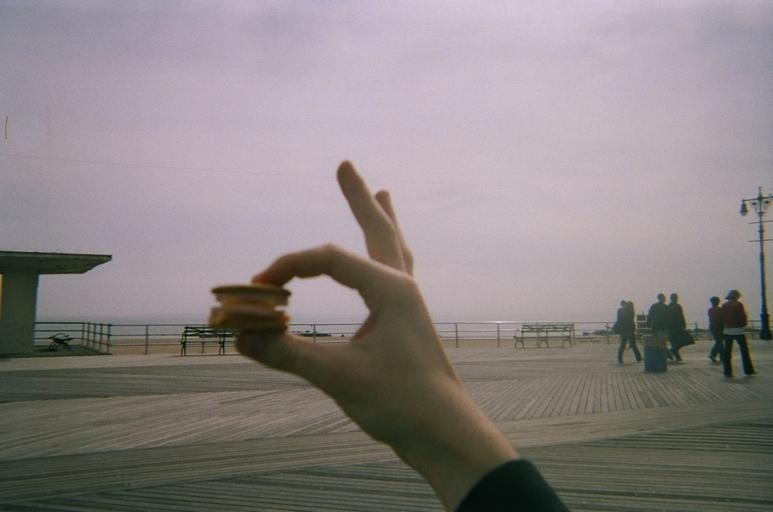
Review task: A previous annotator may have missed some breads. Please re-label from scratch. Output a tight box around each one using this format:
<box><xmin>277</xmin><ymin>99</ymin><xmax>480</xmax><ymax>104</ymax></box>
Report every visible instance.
<box><xmin>206</xmin><ymin>282</ymin><xmax>291</xmax><ymax>332</ymax></box>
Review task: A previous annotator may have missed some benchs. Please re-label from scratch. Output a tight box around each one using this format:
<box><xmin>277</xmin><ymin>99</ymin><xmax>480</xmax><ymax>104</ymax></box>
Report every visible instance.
<box><xmin>179</xmin><ymin>327</ymin><xmax>243</xmax><ymax>356</ymax></box>
<box><xmin>513</xmin><ymin>323</ymin><xmax>575</xmax><ymax>349</ymax></box>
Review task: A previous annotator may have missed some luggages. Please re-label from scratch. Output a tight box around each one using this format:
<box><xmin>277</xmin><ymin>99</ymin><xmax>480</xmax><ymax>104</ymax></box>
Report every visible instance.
<box><xmin>645</xmin><ymin>345</ymin><xmax>667</xmax><ymax>371</ymax></box>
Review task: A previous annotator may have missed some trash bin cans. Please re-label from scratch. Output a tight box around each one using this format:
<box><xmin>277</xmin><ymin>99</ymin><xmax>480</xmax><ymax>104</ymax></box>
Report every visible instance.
<box><xmin>642</xmin><ymin>335</ymin><xmax>668</xmax><ymax>372</ymax></box>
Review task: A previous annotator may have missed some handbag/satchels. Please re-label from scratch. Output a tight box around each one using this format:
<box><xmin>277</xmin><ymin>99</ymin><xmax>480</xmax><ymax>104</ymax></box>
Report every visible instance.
<box><xmin>671</xmin><ymin>328</ymin><xmax>695</xmax><ymax>350</ymax></box>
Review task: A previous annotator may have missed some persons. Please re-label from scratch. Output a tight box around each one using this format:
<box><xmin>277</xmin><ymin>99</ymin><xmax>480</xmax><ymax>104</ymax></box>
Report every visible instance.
<box><xmin>707</xmin><ymin>297</ymin><xmax>724</xmax><ymax>363</ymax></box>
<box><xmin>234</xmin><ymin>160</ymin><xmax>572</xmax><ymax>512</ymax></box>
<box><xmin>719</xmin><ymin>290</ymin><xmax>758</xmax><ymax>377</ymax></box>
<box><xmin>665</xmin><ymin>293</ymin><xmax>686</xmax><ymax>360</ymax></box>
<box><xmin>646</xmin><ymin>293</ymin><xmax>674</xmax><ymax>361</ymax></box>
<box><xmin>615</xmin><ymin>301</ymin><xmax>643</xmax><ymax>366</ymax></box>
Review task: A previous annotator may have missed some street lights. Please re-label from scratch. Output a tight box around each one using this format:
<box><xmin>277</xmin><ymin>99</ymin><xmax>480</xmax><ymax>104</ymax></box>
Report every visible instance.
<box><xmin>740</xmin><ymin>186</ymin><xmax>773</xmax><ymax>340</ymax></box>
